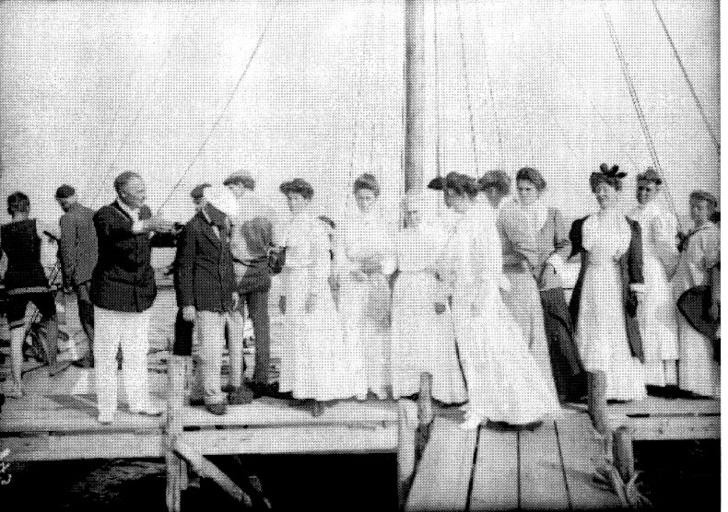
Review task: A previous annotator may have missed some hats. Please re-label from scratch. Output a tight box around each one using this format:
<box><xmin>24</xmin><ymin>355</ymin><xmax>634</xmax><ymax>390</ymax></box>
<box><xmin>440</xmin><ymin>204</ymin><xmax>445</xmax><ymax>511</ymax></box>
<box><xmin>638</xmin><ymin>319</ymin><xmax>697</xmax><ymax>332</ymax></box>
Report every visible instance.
<box><xmin>203</xmin><ymin>186</ymin><xmax>239</xmax><ymax>217</ymax></box>
<box><xmin>56</xmin><ymin>185</ymin><xmax>75</xmax><ymax>197</ymax></box>
<box><xmin>280</xmin><ymin>177</ymin><xmax>315</xmax><ymax>195</ymax></box>
<box><xmin>637</xmin><ymin>168</ymin><xmax>663</xmax><ymax>184</ymax></box>
<box><xmin>224</xmin><ymin>169</ymin><xmax>251</xmax><ymax>185</ymax></box>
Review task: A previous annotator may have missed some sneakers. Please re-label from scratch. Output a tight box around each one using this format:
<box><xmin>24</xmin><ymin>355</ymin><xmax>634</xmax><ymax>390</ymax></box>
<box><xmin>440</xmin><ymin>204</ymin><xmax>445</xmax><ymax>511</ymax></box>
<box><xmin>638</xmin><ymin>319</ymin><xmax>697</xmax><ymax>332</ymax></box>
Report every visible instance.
<box><xmin>97</xmin><ymin>411</ymin><xmax>115</xmax><ymax>424</ymax></box>
<box><xmin>141</xmin><ymin>404</ymin><xmax>163</xmax><ymax>416</ymax></box>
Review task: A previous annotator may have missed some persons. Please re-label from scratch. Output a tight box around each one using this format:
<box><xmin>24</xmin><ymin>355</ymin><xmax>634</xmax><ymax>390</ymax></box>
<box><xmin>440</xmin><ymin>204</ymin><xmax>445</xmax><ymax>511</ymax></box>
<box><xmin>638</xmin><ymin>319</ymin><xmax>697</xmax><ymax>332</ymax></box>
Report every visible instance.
<box><xmin>281</xmin><ymin>162</ymin><xmax>721</xmax><ymax>430</ymax></box>
<box><xmin>52</xmin><ymin>182</ymin><xmax>124</xmax><ymax>372</ymax></box>
<box><xmin>87</xmin><ymin>170</ymin><xmax>182</xmax><ymax>424</ymax></box>
<box><xmin>174</xmin><ymin>180</ymin><xmax>214</xmax><ymax>355</ymax></box>
<box><xmin>1</xmin><ymin>189</ymin><xmax>74</xmax><ymax>400</ymax></box>
<box><xmin>177</xmin><ymin>181</ymin><xmax>254</xmax><ymax>410</ymax></box>
<box><xmin>219</xmin><ymin>167</ymin><xmax>287</xmax><ymax>398</ymax></box>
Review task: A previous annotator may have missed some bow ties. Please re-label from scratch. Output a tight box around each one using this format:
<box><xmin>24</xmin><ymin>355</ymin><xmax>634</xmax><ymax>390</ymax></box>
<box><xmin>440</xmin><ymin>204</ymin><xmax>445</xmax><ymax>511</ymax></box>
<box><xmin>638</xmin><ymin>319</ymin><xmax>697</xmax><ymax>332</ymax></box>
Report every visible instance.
<box><xmin>210</xmin><ymin>221</ymin><xmax>225</xmax><ymax>231</ymax></box>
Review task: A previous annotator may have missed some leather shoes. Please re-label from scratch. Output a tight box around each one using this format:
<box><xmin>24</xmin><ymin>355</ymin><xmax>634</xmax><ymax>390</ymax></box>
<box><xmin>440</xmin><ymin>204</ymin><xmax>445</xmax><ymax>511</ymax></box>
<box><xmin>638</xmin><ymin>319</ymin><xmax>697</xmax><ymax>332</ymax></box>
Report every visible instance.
<box><xmin>290</xmin><ymin>397</ymin><xmax>324</xmax><ymax>417</ymax></box>
<box><xmin>190</xmin><ymin>392</ymin><xmax>226</xmax><ymax>415</ymax></box>
